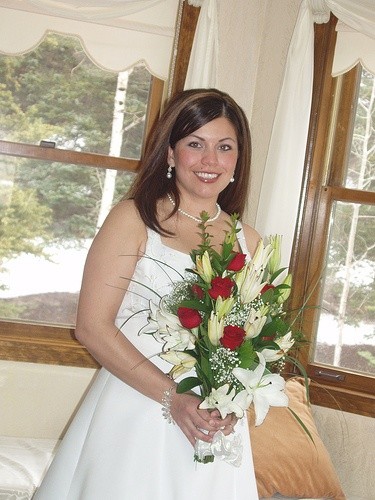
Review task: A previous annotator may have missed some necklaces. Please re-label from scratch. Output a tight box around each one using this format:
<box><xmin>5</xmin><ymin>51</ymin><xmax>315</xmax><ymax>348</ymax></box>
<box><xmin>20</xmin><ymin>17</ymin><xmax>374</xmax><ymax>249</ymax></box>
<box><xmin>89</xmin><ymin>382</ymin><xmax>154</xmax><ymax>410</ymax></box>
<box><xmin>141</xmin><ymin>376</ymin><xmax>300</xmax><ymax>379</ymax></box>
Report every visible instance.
<box><xmin>167</xmin><ymin>190</ymin><xmax>222</xmax><ymax>222</ymax></box>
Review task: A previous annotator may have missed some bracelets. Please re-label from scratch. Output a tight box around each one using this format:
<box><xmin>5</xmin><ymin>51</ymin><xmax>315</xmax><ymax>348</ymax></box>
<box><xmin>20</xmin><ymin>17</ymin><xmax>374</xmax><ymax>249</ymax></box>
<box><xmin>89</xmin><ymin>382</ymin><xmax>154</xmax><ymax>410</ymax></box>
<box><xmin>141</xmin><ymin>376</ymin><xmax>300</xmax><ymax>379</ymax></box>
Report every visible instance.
<box><xmin>162</xmin><ymin>383</ymin><xmax>179</xmax><ymax>424</ymax></box>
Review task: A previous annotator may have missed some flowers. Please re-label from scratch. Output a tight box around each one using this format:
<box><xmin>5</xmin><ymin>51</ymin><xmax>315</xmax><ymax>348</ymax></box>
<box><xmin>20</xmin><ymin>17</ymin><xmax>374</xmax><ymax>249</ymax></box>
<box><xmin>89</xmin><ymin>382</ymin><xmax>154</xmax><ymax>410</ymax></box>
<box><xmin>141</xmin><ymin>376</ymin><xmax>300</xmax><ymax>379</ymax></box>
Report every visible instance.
<box><xmin>98</xmin><ymin>209</ymin><xmax>347</xmax><ymax>464</ymax></box>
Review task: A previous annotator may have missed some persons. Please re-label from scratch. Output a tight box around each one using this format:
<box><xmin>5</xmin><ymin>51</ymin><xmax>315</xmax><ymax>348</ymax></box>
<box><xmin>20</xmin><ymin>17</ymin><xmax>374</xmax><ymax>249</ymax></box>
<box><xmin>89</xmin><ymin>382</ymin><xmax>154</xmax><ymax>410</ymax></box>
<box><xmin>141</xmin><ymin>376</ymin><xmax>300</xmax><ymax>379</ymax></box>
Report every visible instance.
<box><xmin>34</xmin><ymin>88</ymin><xmax>267</xmax><ymax>500</ymax></box>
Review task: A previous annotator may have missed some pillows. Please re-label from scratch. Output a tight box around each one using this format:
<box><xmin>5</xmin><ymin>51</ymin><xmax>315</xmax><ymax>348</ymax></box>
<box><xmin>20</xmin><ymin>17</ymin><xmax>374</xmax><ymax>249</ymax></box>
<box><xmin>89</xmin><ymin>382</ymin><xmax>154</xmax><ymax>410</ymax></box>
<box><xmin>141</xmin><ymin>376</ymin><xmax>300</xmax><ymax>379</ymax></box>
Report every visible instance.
<box><xmin>246</xmin><ymin>373</ymin><xmax>345</xmax><ymax>500</ymax></box>
